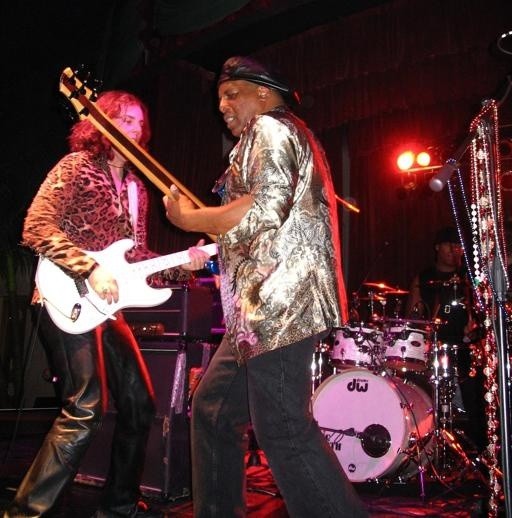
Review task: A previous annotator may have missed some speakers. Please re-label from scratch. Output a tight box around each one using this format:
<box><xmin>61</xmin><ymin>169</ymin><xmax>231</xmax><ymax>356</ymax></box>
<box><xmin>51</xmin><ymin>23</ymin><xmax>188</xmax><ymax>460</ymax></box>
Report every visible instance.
<box><xmin>74</xmin><ymin>340</ymin><xmax>220</xmax><ymax>502</ymax></box>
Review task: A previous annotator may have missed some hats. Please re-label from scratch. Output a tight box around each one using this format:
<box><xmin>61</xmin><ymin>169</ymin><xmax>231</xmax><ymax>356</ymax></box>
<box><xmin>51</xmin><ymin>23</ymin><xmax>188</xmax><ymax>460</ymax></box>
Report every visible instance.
<box><xmin>217</xmin><ymin>56</ymin><xmax>289</xmax><ymax>92</ymax></box>
<box><xmin>433</xmin><ymin>226</ymin><xmax>458</xmax><ymax>244</ymax></box>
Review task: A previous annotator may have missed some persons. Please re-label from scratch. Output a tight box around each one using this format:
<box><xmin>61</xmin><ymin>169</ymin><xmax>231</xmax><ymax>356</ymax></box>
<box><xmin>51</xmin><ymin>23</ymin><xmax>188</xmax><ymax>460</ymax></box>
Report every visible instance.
<box><xmin>405</xmin><ymin>228</ymin><xmax>477</xmax><ymax>458</ymax></box>
<box><xmin>0</xmin><ymin>91</ymin><xmax>209</xmax><ymax>517</ymax></box>
<box><xmin>163</xmin><ymin>58</ymin><xmax>367</xmax><ymax>517</ymax></box>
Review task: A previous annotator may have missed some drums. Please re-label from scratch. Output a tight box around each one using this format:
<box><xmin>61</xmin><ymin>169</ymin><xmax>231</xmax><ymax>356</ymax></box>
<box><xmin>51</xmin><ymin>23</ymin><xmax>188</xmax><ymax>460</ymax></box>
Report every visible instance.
<box><xmin>451</xmin><ymin>345</ymin><xmax>460</xmax><ymax>386</ymax></box>
<box><xmin>308</xmin><ymin>368</ymin><xmax>439</xmax><ymax>483</ymax></box>
<box><xmin>328</xmin><ymin>320</ymin><xmax>384</xmax><ymax>372</ymax></box>
<box><xmin>431</xmin><ymin>341</ymin><xmax>451</xmax><ymax>384</ymax></box>
<box><xmin>382</xmin><ymin>318</ymin><xmax>431</xmax><ymax>372</ymax></box>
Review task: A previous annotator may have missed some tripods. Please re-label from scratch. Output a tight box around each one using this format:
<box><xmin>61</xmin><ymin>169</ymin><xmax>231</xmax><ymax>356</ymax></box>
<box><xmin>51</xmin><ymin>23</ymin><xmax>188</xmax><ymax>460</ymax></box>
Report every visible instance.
<box><xmin>407</xmin><ymin>318</ymin><xmax>482</xmax><ymax>503</ymax></box>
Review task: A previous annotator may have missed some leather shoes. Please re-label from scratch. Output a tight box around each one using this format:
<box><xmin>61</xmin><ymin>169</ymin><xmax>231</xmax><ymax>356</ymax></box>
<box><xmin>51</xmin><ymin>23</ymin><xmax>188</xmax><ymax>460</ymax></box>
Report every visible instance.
<box><xmin>95</xmin><ymin>500</ymin><xmax>157</xmax><ymax>518</ymax></box>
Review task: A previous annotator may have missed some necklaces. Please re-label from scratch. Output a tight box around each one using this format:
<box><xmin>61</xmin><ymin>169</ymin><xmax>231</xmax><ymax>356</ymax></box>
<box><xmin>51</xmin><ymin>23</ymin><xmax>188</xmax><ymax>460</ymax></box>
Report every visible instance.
<box><xmin>109</xmin><ymin>164</ymin><xmax>124</xmax><ymax>168</ymax></box>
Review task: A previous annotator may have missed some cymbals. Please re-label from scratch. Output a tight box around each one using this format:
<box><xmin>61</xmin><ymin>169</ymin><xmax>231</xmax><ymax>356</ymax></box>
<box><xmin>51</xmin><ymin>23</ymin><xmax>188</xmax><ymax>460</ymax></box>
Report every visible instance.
<box><xmin>364</xmin><ymin>281</ymin><xmax>395</xmax><ymax>291</ymax></box>
<box><xmin>383</xmin><ymin>289</ymin><xmax>408</xmax><ymax>297</ymax></box>
<box><xmin>359</xmin><ymin>295</ymin><xmax>387</xmax><ymax>300</ymax></box>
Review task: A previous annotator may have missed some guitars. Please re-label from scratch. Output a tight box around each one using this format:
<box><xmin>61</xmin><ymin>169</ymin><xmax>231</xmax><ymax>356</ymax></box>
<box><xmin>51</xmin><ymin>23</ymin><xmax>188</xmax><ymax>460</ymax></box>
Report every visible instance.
<box><xmin>34</xmin><ymin>239</ymin><xmax>219</xmax><ymax>334</ymax></box>
<box><xmin>58</xmin><ymin>63</ymin><xmax>222</xmax><ymax>290</ymax></box>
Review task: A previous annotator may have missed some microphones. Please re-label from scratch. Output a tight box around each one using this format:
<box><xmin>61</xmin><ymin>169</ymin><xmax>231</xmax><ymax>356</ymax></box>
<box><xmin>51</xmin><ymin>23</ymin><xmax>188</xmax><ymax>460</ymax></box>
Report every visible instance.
<box><xmin>429</xmin><ymin>121</ymin><xmax>482</xmax><ymax>192</ymax></box>
